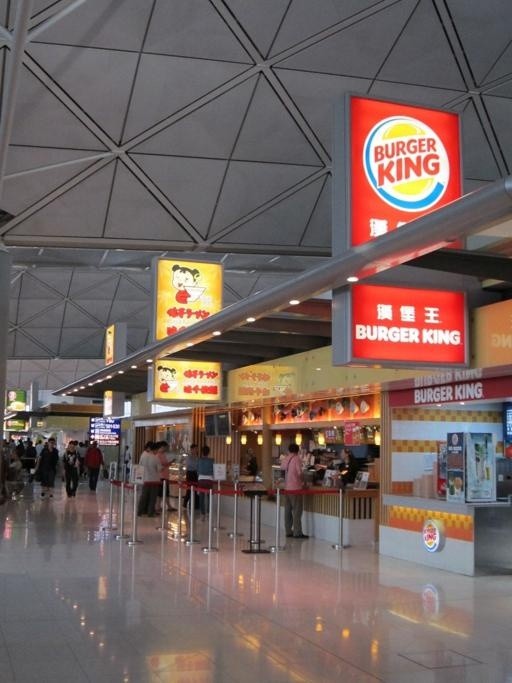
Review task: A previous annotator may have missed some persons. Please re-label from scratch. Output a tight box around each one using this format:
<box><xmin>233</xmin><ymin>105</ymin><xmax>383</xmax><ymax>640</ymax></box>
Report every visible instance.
<box><xmin>317</xmin><ymin>447</ymin><xmax>358</xmax><ymax>486</ymax></box>
<box><xmin>136</xmin><ymin>439</ymin><xmax>215</xmax><ymax>520</ymax></box>
<box><xmin>243</xmin><ymin>446</ymin><xmax>258</xmax><ymax>477</ymax></box>
<box><xmin>1</xmin><ymin>433</ymin><xmax>132</xmax><ymax>499</ymax></box>
<box><xmin>281</xmin><ymin>444</ymin><xmax>310</xmax><ymax>539</ymax></box>
